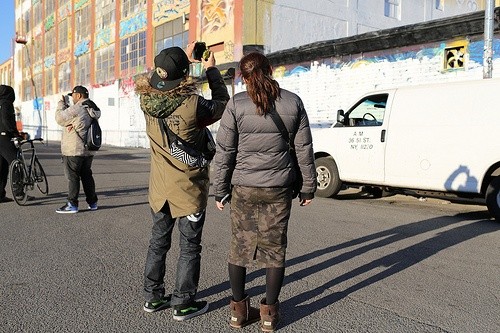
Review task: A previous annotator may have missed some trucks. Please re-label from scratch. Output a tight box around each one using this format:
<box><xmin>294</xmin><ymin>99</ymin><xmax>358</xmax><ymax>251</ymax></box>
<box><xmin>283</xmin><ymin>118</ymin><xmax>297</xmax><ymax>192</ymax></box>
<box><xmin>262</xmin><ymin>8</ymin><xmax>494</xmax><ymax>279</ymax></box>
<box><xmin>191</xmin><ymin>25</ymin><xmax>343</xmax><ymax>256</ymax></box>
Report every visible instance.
<box><xmin>305</xmin><ymin>77</ymin><xmax>500</xmax><ymax>222</ymax></box>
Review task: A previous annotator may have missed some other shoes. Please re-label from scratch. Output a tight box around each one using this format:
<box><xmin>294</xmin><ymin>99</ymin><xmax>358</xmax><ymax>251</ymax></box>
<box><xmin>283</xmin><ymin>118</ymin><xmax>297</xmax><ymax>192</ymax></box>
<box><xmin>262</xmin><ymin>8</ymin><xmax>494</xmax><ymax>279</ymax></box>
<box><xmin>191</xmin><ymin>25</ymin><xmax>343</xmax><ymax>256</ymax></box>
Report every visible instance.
<box><xmin>0</xmin><ymin>196</ymin><xmax>15</xmax><ymax>203</ymax></box>
<box><xmin>15</xmin><ymin>192</ymin><xmax>34</xmax><ymax>200</ymax></box>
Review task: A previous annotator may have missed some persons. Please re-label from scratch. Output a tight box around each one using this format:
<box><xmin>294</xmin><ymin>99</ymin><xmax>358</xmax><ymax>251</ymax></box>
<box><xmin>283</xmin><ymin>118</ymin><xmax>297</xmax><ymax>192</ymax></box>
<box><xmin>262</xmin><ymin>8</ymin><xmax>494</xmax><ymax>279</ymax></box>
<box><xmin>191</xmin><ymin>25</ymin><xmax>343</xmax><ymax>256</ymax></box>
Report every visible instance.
<box><xmin>55</xmin><ymin>86</ymin><xmax>101</xmax><ymax>213</ymax></box>
<box><xmin>0</xmin><ymin>85</ymin><xmax>35</xmax><ymax>203</ymax></box>
<box><xmin>134</xmin><ymin>41</ymin><xmax>230</xmax><ymax>321</ymax></box>
<box><xmin>213</xmin><ymin>52</ymin><xmax>318</xmax><ymax>331</ymax></box>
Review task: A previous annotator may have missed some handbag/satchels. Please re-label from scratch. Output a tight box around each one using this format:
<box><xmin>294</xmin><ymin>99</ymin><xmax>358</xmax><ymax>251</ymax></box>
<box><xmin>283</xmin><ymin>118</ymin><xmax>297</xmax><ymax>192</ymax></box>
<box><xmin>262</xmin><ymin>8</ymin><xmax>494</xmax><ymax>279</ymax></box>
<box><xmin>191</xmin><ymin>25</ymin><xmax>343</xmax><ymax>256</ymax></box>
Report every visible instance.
<box><xmin>168</xmin><ymin>133</ymin><xmax>215</xmax><ymax>166</ymax></box>
<box><xmin>288</xmin><ymin>150</ymin><xmax>304</xmax><ymax>199</ymax></box>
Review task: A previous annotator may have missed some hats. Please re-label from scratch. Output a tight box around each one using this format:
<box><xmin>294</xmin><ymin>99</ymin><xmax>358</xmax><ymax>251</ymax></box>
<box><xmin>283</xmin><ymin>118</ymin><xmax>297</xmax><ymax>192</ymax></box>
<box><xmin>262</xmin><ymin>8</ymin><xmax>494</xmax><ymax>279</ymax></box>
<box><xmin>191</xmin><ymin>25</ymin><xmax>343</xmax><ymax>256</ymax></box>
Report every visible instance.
<box><xmin>68</xmin><ymin>86</ymin><xmax>89</xmax><ymax>96</ymax></box>
<box><xmin>149</xmin><ymin>46</ymin><xmax>189</xmax><ymax>91</ymax></box>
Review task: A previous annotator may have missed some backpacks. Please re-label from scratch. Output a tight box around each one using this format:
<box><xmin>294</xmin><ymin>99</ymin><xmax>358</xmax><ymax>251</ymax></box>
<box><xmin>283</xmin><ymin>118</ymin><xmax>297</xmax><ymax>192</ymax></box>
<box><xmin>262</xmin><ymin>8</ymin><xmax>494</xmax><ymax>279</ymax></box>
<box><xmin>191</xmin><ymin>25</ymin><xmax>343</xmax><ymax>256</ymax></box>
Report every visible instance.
<box><xmin>72</xmin><ymin>106</ymin><xmax>102</xmax><ymax>151</ymax></box>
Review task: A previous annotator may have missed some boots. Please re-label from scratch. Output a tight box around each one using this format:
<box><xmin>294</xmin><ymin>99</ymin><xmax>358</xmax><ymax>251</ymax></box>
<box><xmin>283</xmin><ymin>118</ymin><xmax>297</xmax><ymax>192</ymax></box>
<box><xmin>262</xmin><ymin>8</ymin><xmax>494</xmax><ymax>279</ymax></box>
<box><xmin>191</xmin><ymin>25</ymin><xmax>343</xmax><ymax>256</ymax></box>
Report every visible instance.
<box><xmin>229</xmin><ymin>297</ymin><xmax>261</xmax><ymax>328</ymax></box>
<box><xmin>259</xmin><ymin>296</ymin><xmax>280</xmax><ymax>331</ymax></box>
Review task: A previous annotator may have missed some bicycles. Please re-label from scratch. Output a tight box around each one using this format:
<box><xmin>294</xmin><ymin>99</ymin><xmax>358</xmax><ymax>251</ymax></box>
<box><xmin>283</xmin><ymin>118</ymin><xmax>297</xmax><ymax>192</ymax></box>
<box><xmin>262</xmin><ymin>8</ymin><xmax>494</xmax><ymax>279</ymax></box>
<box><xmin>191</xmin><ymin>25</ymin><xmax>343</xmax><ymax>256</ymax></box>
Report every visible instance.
<box><xmin>8</xmin><ymin>136</ymin><xmax>50</xmax><ymax>206</ymax></box>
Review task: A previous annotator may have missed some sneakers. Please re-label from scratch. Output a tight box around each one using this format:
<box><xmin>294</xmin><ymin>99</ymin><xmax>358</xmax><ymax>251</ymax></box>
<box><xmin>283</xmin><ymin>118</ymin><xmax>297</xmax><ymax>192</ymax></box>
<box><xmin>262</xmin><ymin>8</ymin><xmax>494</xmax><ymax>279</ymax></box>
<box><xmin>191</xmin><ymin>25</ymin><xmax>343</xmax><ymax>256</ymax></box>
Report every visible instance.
<box><xmin>55</xmin><ymin>202</ymin><xmax>78</xmax><ymax>213</ymax></box>
<box><xmin>173</xmin><ymin>300</ymin><xmax>208</xmax><ymax>321</ymax></box>
<box><xmin>88</xmin><ymin>202</ymin><xmax>98</xmax><ymax>210</ymax></box>
<box><xmin>143</xmin><ymin>296</ymin><xmax>171</xmax><ymax>313</ymax></box>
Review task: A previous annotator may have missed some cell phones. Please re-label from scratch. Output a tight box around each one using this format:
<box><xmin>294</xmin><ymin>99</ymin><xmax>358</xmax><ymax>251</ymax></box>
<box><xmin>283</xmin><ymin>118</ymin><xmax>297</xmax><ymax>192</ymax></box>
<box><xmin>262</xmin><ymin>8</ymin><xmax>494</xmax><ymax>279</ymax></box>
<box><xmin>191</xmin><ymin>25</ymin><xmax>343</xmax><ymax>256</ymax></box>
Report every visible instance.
<box><xmin>62</xmin><ymin>95</ymin><xmax>66</xmax><ymax>104</ymax></box>
<box><xmin>195</xmin><ymin>42</ymin><xmax>208</xmax><ymax>62</ymax></box>
<box><xmin>221</xmin><ymin>194</ymin><xmax>231</xmax><ymax>206</ymax></box>
